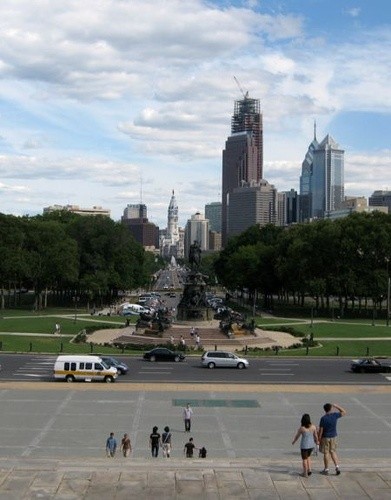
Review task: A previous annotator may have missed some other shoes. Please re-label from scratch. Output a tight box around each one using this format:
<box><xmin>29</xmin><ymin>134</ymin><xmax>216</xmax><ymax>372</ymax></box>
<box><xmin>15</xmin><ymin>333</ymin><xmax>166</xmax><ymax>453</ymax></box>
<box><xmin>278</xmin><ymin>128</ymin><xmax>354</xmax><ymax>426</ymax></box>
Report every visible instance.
<box><xmin>302</xmin><ymin>473</ymin><xmax>308</xmax><ymax>478</ymax></box>
<box><xmin>320</xmin><ymin>469</ymin><xmax>328</xmax><ymax>475</ymax></box>
<box><xmin>308</xmin><ymin>472</ymin><xmax>312</xmax><ymax>475</ymax></box>
<box><xmin>336</xmin><ymin>468</ymin><xmax>340</xmax><ymax>475</ymax></box>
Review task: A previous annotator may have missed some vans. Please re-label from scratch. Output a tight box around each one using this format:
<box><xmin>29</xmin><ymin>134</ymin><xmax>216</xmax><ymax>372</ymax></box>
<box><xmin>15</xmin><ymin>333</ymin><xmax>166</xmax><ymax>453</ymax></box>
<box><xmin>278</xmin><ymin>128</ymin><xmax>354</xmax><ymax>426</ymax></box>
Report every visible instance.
<box><xmin>201</xmin><ymin>351</ymin><xmax>249</xmax><ymax>369</ymax></box>
<box><xmin>53</xmin><ymin>354</ymin><xmax>118</xmax><ymax>383</ymax></box>
<box><xmin>122</xmin><ymin>303</ymin><xmax>149</xmax><ymax>316</ymax></box>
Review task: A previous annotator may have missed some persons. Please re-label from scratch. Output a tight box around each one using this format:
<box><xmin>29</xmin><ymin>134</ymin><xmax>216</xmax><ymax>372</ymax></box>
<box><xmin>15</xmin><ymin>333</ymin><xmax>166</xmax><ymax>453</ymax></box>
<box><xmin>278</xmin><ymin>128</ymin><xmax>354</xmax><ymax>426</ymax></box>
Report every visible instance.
<box><xmin>149</xmin><ymin>426</ymin><xmax>161</xmax><ymax>457</ymax></box>
<box><xmin>198</xmin><ymin>447</ymin><xmax>207</xmax><ymax>458</ymax></box>
<box><xmin>168</xmin><ymin>326</ymin><xmax>201</xmax><ymax>350</ymax></box>
<box><xmin>183</xmin><ymin>403</ymin><xmax>192</xmax><ymax>432</ymax></box>
<box><xmin>53</xmin><ymin>322</ymin><xmax>60</xmax><ymax>334</ymax></box>
<box><xmin>120</xmin><ymin>434</ymin><xmax>132</xmax><ymax>457</ymax></box>
<box><xmin>160</xmin><ymin>426</ymin><xmax>171</xmax><ymax>458</ymax></box>
<box><xmin>183</xmin><ymin>437</ymin><xmax>198</xmax><ymax>458</ymax></box>
<box><xmin>105</xmin><ymin>433</ymin><xmax>116</xmax><ymax>458</ymax></box>
<box><xmin>291</xmin><ymin>413</ymin><xmax>320</xmax><ymax>478</ymax></box>
<box><xmin>318</xmin><ymin>403</ymin><xmax>347</xmax><ymax>475</ymax></box>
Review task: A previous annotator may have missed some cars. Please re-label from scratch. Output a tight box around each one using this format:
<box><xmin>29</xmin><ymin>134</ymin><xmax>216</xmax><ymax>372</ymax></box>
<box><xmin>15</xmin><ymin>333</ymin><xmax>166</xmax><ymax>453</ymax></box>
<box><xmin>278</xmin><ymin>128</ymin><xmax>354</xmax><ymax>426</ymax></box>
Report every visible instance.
<box><xmin>204</xmin><ymin>290</ymin><xmax>234</xmax><ymax>314</ymax></box>
<box><xmin>100</xmin><ymin>356</ymin><xmax>130</xmax><ymax>377</ymax></box>
<box><xmin>143</xmin><ymin>347</ymin><xmax>185</xmax><ymax>361</ymax></box>
<box><xmin>351</xmin><ymin>358</ymin><xmax>391</xmax><ymax>373</ymax></box>
